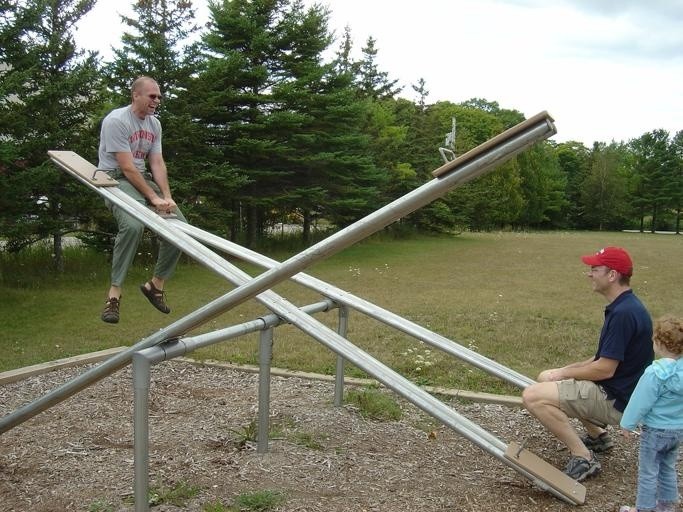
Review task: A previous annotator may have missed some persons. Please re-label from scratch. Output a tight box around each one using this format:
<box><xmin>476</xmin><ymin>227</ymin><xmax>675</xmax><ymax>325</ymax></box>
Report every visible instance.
<box><xmin>520</xmin><ymin>245</ymin><xmax>656</xmax><ymax>482</ymax></box>
<box><xmin>96</xmin><ymin>76</ymin><xmax>189</xmax><ymax>324</ymax></box>
<box><xmin>618</xmin><ymin>313</ymin><xmax>683</xmax><ymax>512</ymax></box>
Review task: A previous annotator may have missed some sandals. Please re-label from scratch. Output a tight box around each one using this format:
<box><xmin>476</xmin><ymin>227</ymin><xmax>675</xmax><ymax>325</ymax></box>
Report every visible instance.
<box><xmin>101</xmin><ymin>295</ymin><xmax>122</xmax><ymax>323</ymax></box>
<box><xmin>141</xmin><ymin>280</ymin><xmax>170</xmax><ymax>314</ymax></box>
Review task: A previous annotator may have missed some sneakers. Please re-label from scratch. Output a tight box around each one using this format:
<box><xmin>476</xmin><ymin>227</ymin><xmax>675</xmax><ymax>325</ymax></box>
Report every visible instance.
<box><xmin>656</xmin><ymin>500</ymin><xmax>675</xmax><ymax>512</ymax></box>
<box><xmin>581</xmin><ymin>434</ymin><xmax>613</xmax><ymax>453</ymax></box>
<box><xmin>562</xmin><ymin>448</ymin><xmax>601</xmax><ymax>482</ymax></box>
<box><xmin>619</xmin><ymin>505</ymin><xmax>639</xmax><ymax>512</ymax></box>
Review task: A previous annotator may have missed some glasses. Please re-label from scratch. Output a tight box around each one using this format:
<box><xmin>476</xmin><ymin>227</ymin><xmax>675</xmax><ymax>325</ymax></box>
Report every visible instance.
<box><xmin>149</xmin><ymin>94</ymin><xmax>162</xmax><ymax>99</ymax></box>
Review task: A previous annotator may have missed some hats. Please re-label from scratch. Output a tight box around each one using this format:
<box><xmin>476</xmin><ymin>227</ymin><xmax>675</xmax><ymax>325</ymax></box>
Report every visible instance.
<box><xmin>580</xmin><ymin>246</ymin><xmax>633</xmax><ymax>275</ymax></box>
